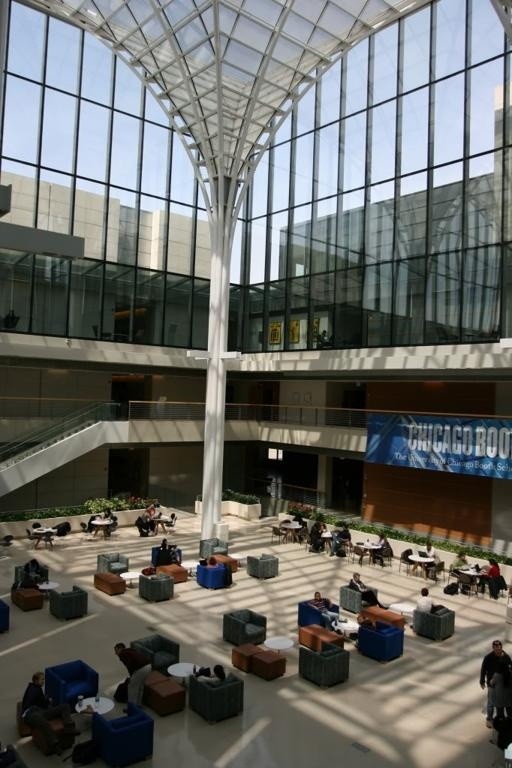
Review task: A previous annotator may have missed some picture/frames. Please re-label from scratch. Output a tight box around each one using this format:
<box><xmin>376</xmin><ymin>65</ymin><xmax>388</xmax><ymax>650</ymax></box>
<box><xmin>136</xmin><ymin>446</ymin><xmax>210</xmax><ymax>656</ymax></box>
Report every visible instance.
<box><xmin>312</xmin><ymin>317</ymin><xmax>320</xmax><ymax>341</ymax></box>
<box><xmin>288</xmin><ymin>319</ymin><xmax>301</xmax><ymax>343</ymax></box>
<box><xmin>268</xmin><ymin>320</ymin><xmax>281</xmax><ymax>345</ymax></box>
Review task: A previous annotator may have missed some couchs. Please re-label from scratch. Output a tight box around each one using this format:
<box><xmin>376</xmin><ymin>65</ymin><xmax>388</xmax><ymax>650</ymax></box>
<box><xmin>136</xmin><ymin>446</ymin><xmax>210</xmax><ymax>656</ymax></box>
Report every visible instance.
<box><xmin>97</xmin><ymin>538</ymin><xmax>233</xmax><ymax>601</ymax></box>
<box><xmin>246</xmin><ymin>553</ymin><xmax>278</xmax><ymax>579</ymax></box>
<box><xmin>412</xmin><ymin>605</ymin><xmax>455</xmax><ymax>642</ymax></box>
<box><xmin>223</xmin><ymin>609</ymin><xmax>267</xmax><ymax>647</ymax></box>
<box><xmin>1</xmin><ymin>563</ymin><xmax>88</xmax><ymax>633</ymax></box>
<box><xmin>1</xmin><ymin>634</ymin><xmax>244</xmax><ymax>767</ymax></box>
<box><xmin>298</xmin><ymin>583</ymin><xmax>404</xmax><ymax>689</ymax></box>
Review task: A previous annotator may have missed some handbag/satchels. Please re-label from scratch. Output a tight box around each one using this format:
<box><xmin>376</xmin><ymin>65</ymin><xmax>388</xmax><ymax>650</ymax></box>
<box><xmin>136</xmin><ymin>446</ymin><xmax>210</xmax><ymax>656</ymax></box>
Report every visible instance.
<box><xmin>113</xmin><ymin>681</ymin><xmax>128</xmax><ymax>704</ymax></box>
<box><xmin>71</xmin><ymin>739</ymin><xmax>101</xmax><ymax>763</ymax></box>
<box><xmin>336</xmin><ymin>550</ymin><xmax>346</xmax><ymax>557</ymax></box>
<box><xmin>444</xmin><ymin>583</ymin><xmax>459</xmax><ymax>595</ymax></box>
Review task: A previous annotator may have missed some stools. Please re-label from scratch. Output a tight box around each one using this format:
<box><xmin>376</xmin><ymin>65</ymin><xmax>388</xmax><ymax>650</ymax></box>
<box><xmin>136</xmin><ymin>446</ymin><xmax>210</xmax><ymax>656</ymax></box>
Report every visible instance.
<box><xmin>213</xmin><ymin>554</ymin><xmax>237</xmax><ymax>572</ymax></box>
<box><xmin>94</xmin><ymin>572</ymin><xmax>126</xmax><ymax>596</ymax></box>
<box><xmin>232</xmin><ymin>644</ymin><xmax>287</xmax><ymax>682</ymax></box>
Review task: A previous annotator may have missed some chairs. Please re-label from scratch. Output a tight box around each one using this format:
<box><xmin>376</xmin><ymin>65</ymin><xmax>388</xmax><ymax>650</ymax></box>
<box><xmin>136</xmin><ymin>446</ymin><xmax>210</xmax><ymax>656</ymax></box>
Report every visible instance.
<box><xmin>136</xmin><ymin>512</ymin><xmax>177</xmax><ymax>535</ymax></box>
<box><xmin>435</xmin><ymin>326</ymin><xmax>458</xmax><ymax>344</ymax></box>
<box><xmin>479</xmin><ymin>323</ymin><xmax>499</xmax><ymax>340</ymax></box>
<box><xmin>132</xmin><ymin>329</ymin><xmax>146</xmax><ymax>342</ymax></box>
<box><xmin>399</xmin><ymin>548</ymin><xmax>446</xmax><ymax>584</ymax></box>
<box><xmin>80</xmin><ymin>515</ymin><xmax>118</xmax><ymax>538</ymax></box>
<box><xmin>26</xmin><ymin>521</ymin><xmax>71</xmax><ymax>549</ymax></box>
<box><xmin>449</xmin><ymin>560</ymin><xmax>505</xmax><ymax>600</ymax></box>
<box><xmin>2</xmin><ymin>535</ymin><xmax>14</xmax><ymax>547</ymax></box>
<box><xmin>1</xmin><ymin>315</ymin><xmax>21</xmax><ymax>330</ymax></box>
<box><xmin>92</xmin><ymin>324</ymin><xmax>112</xmax><ymax>340</ymax></box>
<box><xmin>273</xmin><ymin>519</ymin><xmax>394</xmax><ymax>569</ymax></box>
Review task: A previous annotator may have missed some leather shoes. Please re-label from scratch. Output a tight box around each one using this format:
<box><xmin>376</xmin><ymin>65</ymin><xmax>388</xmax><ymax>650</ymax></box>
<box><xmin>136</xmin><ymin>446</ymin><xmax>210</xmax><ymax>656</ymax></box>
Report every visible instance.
<box><xmin>384</xmin><ymin>607</ymin><xmax>390</xmax><ymax>610</ymax></box>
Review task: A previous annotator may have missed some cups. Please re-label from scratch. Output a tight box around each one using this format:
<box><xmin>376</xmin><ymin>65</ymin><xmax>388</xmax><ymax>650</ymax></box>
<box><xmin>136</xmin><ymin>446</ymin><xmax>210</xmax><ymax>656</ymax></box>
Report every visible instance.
<box><xmin>77</xmin><ymin>695</ymin><xmax>84</xmax><ymax>707</ymax></box>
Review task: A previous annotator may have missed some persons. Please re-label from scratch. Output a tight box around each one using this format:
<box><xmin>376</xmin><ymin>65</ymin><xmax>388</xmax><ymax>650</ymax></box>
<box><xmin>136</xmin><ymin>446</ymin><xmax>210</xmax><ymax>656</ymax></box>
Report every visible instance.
<box><xmin>321</xmin><ymin>330</ymin><xmax>333</xmax><ymax>346</ymax></box>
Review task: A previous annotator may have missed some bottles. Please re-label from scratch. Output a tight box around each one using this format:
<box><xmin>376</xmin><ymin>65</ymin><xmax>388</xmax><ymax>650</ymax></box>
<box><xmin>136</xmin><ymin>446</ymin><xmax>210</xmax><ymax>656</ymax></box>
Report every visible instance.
<box><xmin>95</xmin><ymin>691</ymin><xmax>101</xmax><ymax>708</ymax></box>
<box><xmin>334</xmin><ymin>614</ymin><xmax>338</xmax><ymax>627</ymax></box>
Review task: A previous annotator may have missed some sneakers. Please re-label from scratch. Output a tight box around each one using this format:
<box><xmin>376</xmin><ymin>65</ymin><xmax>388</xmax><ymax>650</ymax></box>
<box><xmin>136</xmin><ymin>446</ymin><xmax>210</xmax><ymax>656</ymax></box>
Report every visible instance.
<box><xmin>486</xmin><ymin>719</ymin><xmax>493</xmax><ymax>728</ymax></box>
<box><xmin>462</xmin><ymin>582</ymin><xmax>485</xmax><ymax>595</ymax></box>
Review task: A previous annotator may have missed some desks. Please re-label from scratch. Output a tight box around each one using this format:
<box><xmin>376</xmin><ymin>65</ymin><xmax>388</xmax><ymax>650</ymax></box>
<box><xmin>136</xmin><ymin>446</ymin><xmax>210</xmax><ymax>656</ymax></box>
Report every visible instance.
<box><xmin>111</xmin><ymin>332</ymin><xmax>129</xmax><ymax>342</ymax></box>
<box><xmin>389</xmin><ymin>603</ymin><xmax>415</xmax><ymax>623</ymax></box>
<box><xmin>464</xmin><ymin>332</ymin><xmax>475</xmax><ymax>342</ymax></box>
